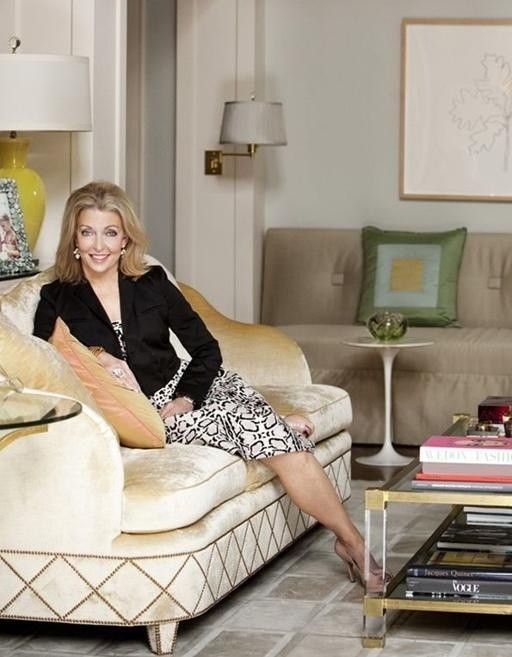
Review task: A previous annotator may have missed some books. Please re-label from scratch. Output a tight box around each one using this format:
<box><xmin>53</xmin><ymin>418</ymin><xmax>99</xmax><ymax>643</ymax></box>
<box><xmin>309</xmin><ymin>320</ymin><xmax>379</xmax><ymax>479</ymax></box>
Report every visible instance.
<box><xmin>402</xmin><ymin>433</ymin><xmax>512</xmax><ymax>602</ymax></box>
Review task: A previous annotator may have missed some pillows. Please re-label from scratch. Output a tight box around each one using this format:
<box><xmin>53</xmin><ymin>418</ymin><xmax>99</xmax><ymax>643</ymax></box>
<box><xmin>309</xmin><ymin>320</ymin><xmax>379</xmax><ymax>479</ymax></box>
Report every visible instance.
<box><xmin>354</xmin><ymin>222</ymin><xmax>467</xmax><ymax>328</ymax></box>
<box><xmin>51</xmin><ymin>315</ymin><xmax>166</xmax><ymax>447</ymax></box>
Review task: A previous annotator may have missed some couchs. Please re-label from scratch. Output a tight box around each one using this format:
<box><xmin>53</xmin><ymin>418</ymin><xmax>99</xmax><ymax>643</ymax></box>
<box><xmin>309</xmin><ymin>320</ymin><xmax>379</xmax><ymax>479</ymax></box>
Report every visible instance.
<box><xmin>0</xmin><ymin>269</ymin><xmax>344</xmax><ymax>655</ymax></box>
<box><xmin>262</xmin><ymin>223</ymin><xmax>511</xmax><ymax>446</ymax></box>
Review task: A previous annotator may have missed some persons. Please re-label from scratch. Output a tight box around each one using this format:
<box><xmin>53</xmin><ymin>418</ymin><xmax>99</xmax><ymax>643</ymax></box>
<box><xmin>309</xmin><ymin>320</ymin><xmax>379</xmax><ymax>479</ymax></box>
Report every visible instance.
<box><xmin>0</xmin><ymin>211</ymin><xmax>21</xmax><ymax>258</ymax></box>
<box><xmin>32</xmin><ymin>178</ymin><xmax>401</xmax><ymax>595</ymax></box>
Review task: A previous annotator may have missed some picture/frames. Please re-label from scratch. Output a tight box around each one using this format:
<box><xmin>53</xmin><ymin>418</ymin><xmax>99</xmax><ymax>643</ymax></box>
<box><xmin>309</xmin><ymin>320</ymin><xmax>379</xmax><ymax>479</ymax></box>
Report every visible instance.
<box><xmin>396</xmin><ymin>15</ymin><xmax>512</xmax><ymax>206</ymax></box>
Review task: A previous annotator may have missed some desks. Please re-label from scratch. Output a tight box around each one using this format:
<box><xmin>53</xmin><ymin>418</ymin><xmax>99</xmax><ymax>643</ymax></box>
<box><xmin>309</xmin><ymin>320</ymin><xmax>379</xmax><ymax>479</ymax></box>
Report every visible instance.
<box><xmin>359</xmin><ymin>413</ymin><xmax>512</xmax><ymax>648</ymax></box>
<box><xmin>339</xmin><ymin>336</ymin><xmax>435</xmax><ymax>466</ymax></box>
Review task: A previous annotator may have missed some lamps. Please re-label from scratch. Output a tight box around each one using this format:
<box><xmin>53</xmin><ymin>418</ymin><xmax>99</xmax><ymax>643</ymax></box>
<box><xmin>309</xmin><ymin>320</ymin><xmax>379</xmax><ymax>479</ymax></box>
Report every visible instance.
<box><xmin>196</xmin><ymin>99</ymin><xmax>289</xmax><ymax>180</ymax></box>
<box><xmin>0</xmin><ymin>52</ymin><xmax>102</xmax><ymax>141</ymax></box>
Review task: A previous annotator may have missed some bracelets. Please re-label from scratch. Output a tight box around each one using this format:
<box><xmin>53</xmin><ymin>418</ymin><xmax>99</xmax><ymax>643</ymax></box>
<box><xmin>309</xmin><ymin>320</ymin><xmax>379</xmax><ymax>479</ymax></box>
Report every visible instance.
<box><xmin>176</xmin><ymin>394</ymin><xmax>197</xmax><ymax>406</ymax></box>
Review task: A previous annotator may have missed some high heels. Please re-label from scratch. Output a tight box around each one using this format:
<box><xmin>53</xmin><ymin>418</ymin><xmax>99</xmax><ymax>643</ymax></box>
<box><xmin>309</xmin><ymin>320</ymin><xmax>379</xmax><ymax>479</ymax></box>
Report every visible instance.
<box><xmin>333</xmin><ymin>539</ymin><xmax>393</xmax><ymax>590</ymax></box>
<box><xmin>282</xmin><ymin>414</ymin><xmax>314</xmax><ymax>438</ymax></box>
